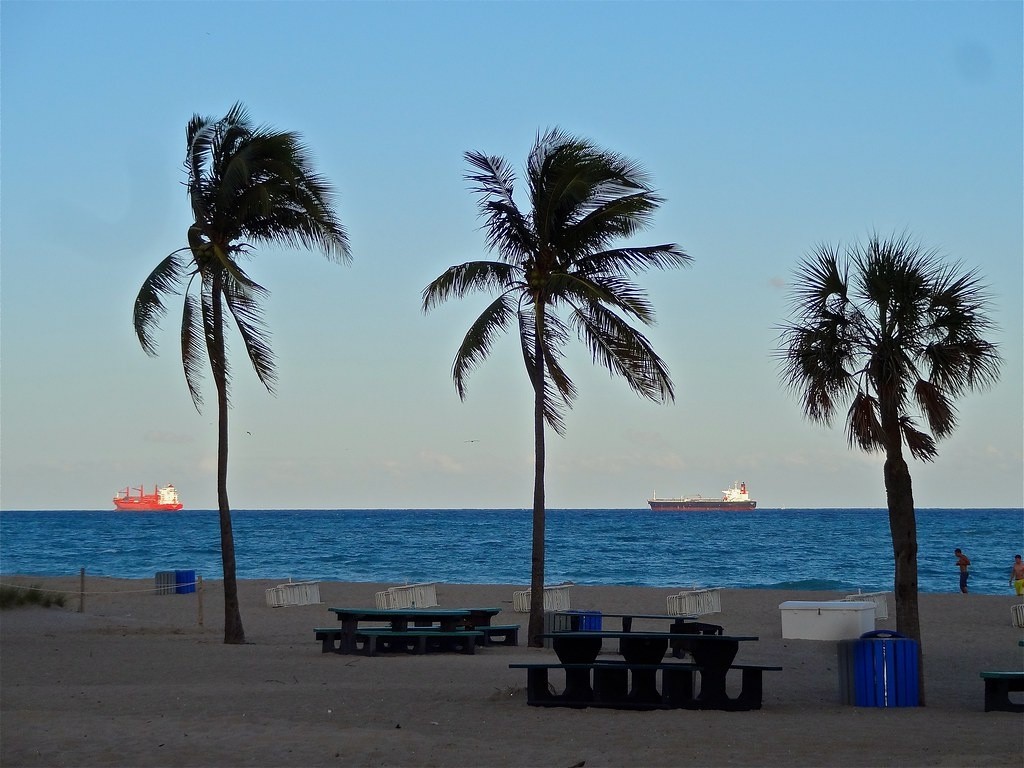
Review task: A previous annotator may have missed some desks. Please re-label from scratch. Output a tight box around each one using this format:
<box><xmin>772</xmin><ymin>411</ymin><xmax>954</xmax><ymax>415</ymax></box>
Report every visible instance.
<box><xmin>534</xmin><ymin>632</ymin><xmax>759</xmax><ymax>699</ymax></box>
<box><xmin>598</xmin><ymin>614</ymin><xmax>698</xmax><ymax>654</ymax></box>
<box><xmin>327</xmin><ymin>607</ymin><xmax>471</xmax><ymax>655</ymax></box>
<box><xmin>389</xmin><ymin>608</ymin><xmax>501</xmax><ymax>646</ymax></box>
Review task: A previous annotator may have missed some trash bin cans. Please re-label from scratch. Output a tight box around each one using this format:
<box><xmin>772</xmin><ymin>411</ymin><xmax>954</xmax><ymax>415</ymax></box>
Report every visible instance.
<box><xmin>546</xmin><ymin>609</ymin><xmax>601</xmax><ymax>649</ymax></box>
<box><xmin>837</xmin><ymin>630</ymin><xmax>920</xmax><ymax>709</ymax></box>
<box><xmin>153</xmin><ymin>569</ymin><xmax>197</xmax><ymax>596</ymax></box>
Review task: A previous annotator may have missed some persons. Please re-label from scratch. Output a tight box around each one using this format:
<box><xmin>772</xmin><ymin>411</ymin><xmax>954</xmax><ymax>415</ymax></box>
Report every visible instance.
<box><xmin>1008</xmin><ymin>554</ymin><xmax>1024</xmax><ymax>596</ymax></box>
<box><xmin>953</xmin><ymin>549</ymin><xmax>972</xmax><ymax>595</ymax></box>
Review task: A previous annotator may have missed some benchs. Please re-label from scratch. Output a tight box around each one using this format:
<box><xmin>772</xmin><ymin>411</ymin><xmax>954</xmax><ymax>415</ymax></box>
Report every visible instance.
<box><xmin>979</xmin><ymin>671</ymin><xmax>1024</xmax><ymax>712</ymax></box>
<box><xmin>591</xmin><ymin>660</ymin><xmax>782</xmax><ymax>710</ymax></box>
<box><xmin>508</xmin><ymin>663</ymin><xmax>704</xmax><ymax>711</ymax></box>
<box><xmin>312</xmin><ymin>626</ymin><xmax>441</xmax><ymax>653</ymax></box>
<box><xmin>432</xmin><ymin>624</ymin><xmax>522</xmax><ymax>647</ymax></box>
<box><xmin>354</xmin><ymin>631</ymin><xmax>484</xmax><ymax>657</ymax></box>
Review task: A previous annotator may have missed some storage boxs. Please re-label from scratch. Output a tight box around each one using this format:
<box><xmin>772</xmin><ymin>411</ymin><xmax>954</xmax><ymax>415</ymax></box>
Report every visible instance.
<box><xmin>778</xmin><ymin>601</ymin><xmax>876</xmax><ymax>640</ymax></box>
<box><xmin>554</xmin><ymin>610</ymin><xmax>602</xmax><ymax>632</ymax></box>
<box><xmin>156</xmin><ymin>571</ymin><xmax>176</xmax><ymax>595</ymax></box>
<box><xmin>176</xmin><ymin>570</ymin><xmax>196</xmax><ymax>594</ymax></box>
<box><xmin>837</xmin><ymin>630</ymin><xmax>919</xmax><ymax>706</ymax></box>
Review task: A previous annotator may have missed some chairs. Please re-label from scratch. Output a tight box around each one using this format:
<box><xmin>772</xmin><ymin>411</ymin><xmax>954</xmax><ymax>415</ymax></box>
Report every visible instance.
<box><xmin>375</xmin><ymin>580</ymin><xmax>440</xmax><ymax>609</ymax></box>
<box><xmin>828</xmin><ymin>591</ymin><xmax>892</xmax><ymax>620</ymax></box>
<box><xmin>1010</xmin><ymin>603</ymin><xmax>1024</xmax><ymax>628</ymax></box>
<box><xmin>265</xmin><ymin>581</ymin><xmax>325</xmax><ymax>608</ymax></box>
<box><xmin>666</xmin><ymin>587</ymin><xmax>725</xmax><ymax>615</ymax></box>
<box><xmin>513</xmin><ymin>584</ymin><xmax>574</xmax><ymax>613</ymax></box>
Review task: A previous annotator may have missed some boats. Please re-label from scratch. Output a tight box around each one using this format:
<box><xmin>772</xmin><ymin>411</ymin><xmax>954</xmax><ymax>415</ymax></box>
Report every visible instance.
<box><xmin>113</xmin><ymin>483</ymin><xmax>184</xmax><ymax>511</ymax></box>
<box><xmin>646</xmin><ymin>480</ymin><xmax>757</xmax><ymax>511</ymax></box>
